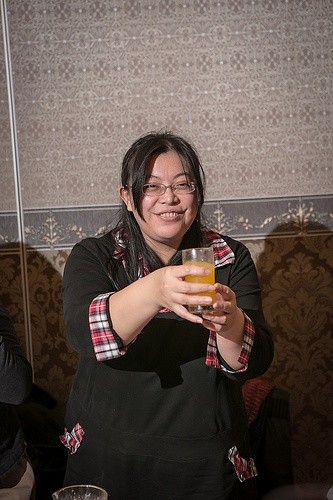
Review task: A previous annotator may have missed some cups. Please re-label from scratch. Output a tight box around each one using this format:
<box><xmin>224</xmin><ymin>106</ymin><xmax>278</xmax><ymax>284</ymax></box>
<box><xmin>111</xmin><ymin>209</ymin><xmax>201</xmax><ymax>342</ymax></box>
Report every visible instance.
<box><xmin>182</xmin><ymin>247</ymin><xmax>216</xmax><ymax>314</ymax></box>
<box><xmin>51</xmin><ymin>485</ymin><xmax>107</xmax><ymax>500</ymax></box>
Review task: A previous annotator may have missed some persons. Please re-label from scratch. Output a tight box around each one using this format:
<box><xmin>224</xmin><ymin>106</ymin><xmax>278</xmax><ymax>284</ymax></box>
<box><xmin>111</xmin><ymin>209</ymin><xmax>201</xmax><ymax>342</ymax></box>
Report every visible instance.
<box><xmin>0</xmin><ymin>314</ymin><xmax>33</xmax><ymax>500</ymax></box>
<box><xmin>59</xmin><ymin>131</ymin><xmax>274</xmax><ymax>500</ymax></box>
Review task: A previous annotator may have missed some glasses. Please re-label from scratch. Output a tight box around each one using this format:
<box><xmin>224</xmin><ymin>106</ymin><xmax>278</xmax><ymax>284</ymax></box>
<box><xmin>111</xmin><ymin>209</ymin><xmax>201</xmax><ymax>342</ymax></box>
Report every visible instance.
<box><xmin>124</xmin><ymin>182</ymin><xmax>198</xmax><ymax>196</ymax></box>
